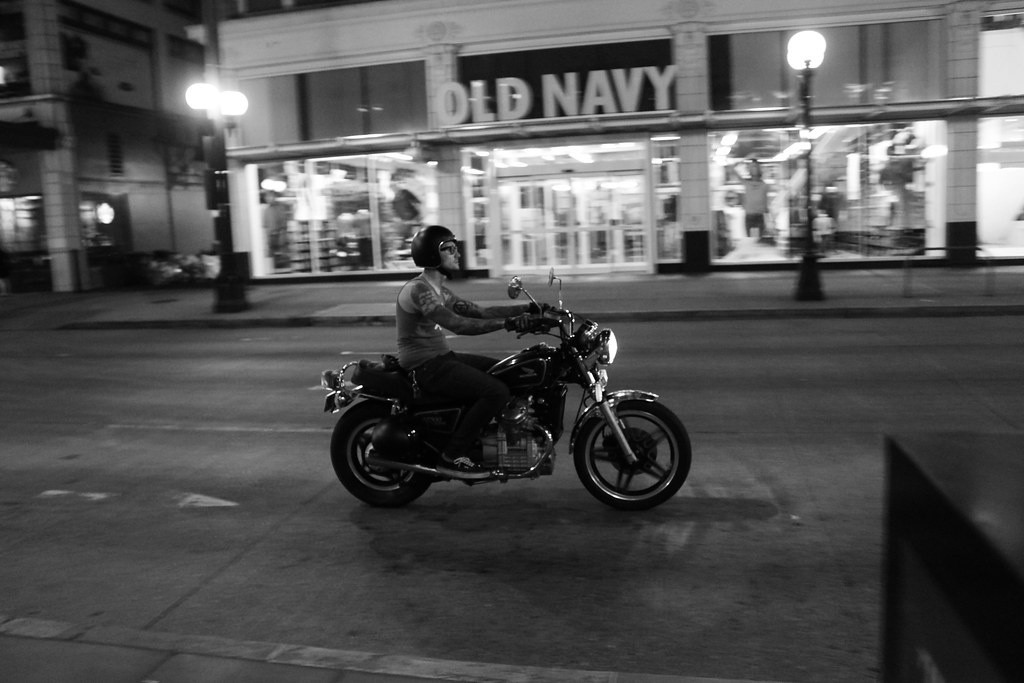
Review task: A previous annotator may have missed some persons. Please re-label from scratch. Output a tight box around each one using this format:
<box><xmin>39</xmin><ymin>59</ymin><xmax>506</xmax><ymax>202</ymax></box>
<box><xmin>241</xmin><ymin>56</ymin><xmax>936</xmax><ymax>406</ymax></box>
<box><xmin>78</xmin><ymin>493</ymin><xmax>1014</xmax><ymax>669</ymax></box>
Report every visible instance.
<box><xmin>396</xmin><ymin>225</ymin><xmax>549</xmax><ymax>472</ymax></box>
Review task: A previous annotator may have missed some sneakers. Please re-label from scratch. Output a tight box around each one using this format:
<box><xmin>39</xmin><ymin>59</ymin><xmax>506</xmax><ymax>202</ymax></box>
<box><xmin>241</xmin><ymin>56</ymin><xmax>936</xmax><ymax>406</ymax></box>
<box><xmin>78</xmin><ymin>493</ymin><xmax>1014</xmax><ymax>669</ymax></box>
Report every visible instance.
<box><xmin>437</xmin><ymin>451</ymin><xmax>490</xmax><ymax>478</ymax></box>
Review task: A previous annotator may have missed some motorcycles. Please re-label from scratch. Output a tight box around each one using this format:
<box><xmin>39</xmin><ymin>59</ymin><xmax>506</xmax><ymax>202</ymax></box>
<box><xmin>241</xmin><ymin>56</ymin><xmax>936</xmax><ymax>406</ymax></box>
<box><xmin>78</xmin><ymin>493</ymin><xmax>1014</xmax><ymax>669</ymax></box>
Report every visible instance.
<box><xmin>318</xmin><ymin>267</ymin><xmax>693</xmax><ymax>511</ymax></box>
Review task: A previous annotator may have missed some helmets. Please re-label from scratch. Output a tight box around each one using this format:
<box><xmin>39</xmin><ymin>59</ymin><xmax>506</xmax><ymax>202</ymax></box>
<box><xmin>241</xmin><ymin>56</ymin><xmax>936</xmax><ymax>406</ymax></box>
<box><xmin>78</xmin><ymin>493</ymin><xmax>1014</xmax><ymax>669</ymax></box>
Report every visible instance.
<box><xmin>411</xmin><ymin>225</ymin><xmax>455</xmax><ymax>268</ymax></box>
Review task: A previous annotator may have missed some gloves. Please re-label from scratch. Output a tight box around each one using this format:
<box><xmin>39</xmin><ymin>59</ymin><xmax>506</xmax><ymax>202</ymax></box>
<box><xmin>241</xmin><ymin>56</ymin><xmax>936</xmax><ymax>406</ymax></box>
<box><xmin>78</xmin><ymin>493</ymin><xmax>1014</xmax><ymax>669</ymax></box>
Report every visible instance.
<box><xmin>504</xmin><ymin>312</ymin><xmax>533</xmax><ymax>332</ymax></box>
<box><xmin>529</xmin><ymin>301</ymin><xmax>549</xmax><ymax>313</ymax></box>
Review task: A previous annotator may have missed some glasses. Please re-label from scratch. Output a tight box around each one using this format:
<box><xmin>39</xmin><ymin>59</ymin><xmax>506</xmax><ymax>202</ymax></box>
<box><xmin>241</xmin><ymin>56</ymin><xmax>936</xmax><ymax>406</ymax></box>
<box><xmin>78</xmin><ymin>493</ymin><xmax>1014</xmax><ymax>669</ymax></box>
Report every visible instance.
<box><xmin>441</xmin><ymin>245</ymin><xmax>459</xmax><ymax>254</ymax></box>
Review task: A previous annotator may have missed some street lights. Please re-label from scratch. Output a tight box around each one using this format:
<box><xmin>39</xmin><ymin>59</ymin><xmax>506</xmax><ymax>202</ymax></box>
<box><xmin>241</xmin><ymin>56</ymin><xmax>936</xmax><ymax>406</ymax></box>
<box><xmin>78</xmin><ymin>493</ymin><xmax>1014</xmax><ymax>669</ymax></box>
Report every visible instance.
<box><xmin>783</xmin><ymin>29</ymin><xmax>827</xmax><ymax>303</ymax></box>
<box><xmin>184</xmin><ymin>82</ymin><xmax>249</xmax><ymax>315</ymax></box>
